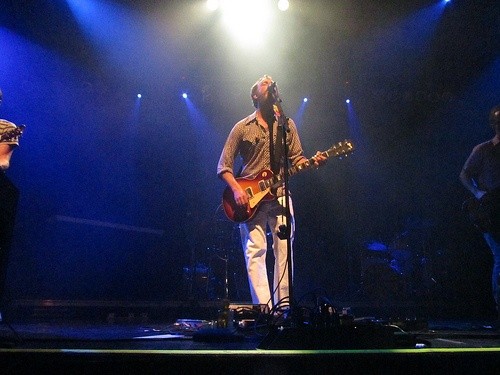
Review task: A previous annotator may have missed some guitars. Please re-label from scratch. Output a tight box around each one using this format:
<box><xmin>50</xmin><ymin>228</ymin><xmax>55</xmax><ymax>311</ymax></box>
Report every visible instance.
<box><xmin>222</xmin><ymin>139</ymin><xmax>353</xmax><ymax>223</ymax></box>
<box><xmin>461</xmin><ymin>186</ymin><xmax>500</xmax><ymax>232</ymax></box>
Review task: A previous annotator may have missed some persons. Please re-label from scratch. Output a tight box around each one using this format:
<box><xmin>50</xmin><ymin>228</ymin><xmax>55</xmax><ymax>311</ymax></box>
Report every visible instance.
<box><xmin>460</xmin><ymin>105</ymin><xmax>500</xmax><ymax>311</ymax></box>
<box><xmin>217</xmin><ymin>75</ymin><xmax>331</xmax><ymax>320</ymax></box>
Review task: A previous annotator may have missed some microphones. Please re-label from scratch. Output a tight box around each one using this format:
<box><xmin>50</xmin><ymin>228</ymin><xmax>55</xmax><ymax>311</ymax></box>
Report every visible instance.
<box><xmin>267</xmin><ymin>82</ymin><xmax>276</xmax><ymax>94</ymax></box>
